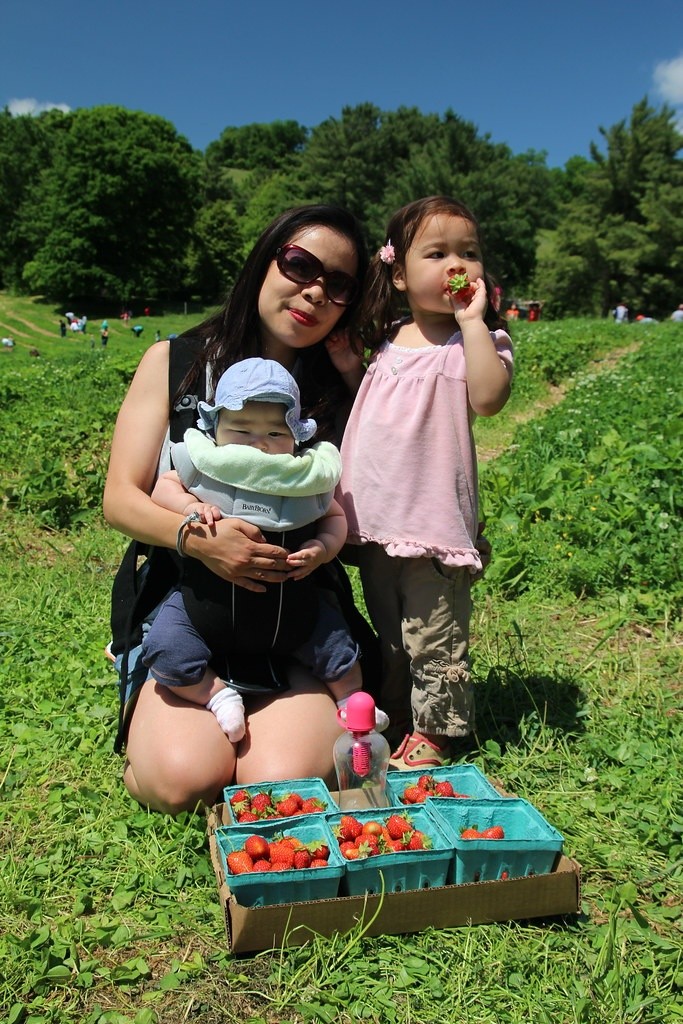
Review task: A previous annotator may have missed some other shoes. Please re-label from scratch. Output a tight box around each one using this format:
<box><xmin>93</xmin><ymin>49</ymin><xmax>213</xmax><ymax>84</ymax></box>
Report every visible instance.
<box><xmin>382</xmin><ymin>715</ymin><xmax>410</xmax><ymax>742</ymax></box>
<box><xmin>389</xmin><ymin>734</ymin><xmax>454</xmax><ymax>771</ymax></box>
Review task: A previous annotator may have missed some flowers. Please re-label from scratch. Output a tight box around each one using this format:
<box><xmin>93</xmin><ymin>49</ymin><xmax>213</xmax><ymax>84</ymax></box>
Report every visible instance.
<box><xmin>379</xmin><ymin>239</ymin><xmax>395</xmax><ymax>265</ymax></box>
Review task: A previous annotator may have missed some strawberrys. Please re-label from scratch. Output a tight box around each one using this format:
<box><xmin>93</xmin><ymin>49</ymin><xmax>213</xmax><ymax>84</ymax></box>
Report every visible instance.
<box><xmin>402</xmin><ymin>775</ymin><xmax>472</xmax><ymax>805</ymax></box>
<box><xmin>226</xmin><ymin>830</ymin><xmax>330</xmax><ymax>875</ymax></box>
<box><xmin>230</xmin><ymin>787</ymin><xmax>327</xmax><ymax>823</ymax></box>
<box><xmin>459</xmin><ymin>824</ymin><xmax>508</xmax><ymax>879</ymax></box>
<box><xmin>447</xmin><ymin>273</ymin><xmax>471</xmax><ymax>297</ymax></box>
<box><xmin>332</xmin><ymin>809</ymin><xmax>434</xmax><ymax>861</ymax></box>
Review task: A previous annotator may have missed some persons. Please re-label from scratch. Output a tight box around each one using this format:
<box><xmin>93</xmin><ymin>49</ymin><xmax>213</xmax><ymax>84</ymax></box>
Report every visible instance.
<box><xmin>506</xmin><ymin>302</ymin><xmax>519</xmax><ymax>321</ymax></box>
<box><xmin>527</xmin><ymin>300</ymin><xmax>542</xmax><ymax>322</ymax></box>
<box><xmin>612</xmin><ymin>304</ymin><xmax>658</xmax><ymax>324</ymax></box>
<box><xmin>102</xmin><ymin>326</ymin><xmax>109</xmax><ymax>345</ymax></box>
<box><xmin>156</xmin><ymin>330</ymin><xmax>178</xmax><ymax>342</ymax></box>
<box><xmin>120</xmin><ymin>311</ymin><xmax>132</xmax><ymax>326</ymax></box>
<box><xmin>140</xmin><ymin>356</ymin><xmax>391</xmax><ymax>743</ymax></box>
<box><xmin>326</xmin><ymin>193</ymin><xmax>516</xmax><ymax>772</ymax></box>
<box><xmin>670</xmin><ymin>303</ymin><xmax>683</xmax><ymax>324</ymax></box>
<box><xmin>130</xmin><ymin>326</ymin><xmax>144</xmax><ymax>337</ymax></box>
<box><xmin>5</xmin><ymin>335</ymin><xmax>16</xmax><ymax>351</ymax></box>
<box><xmin>101</xmin><ymin>200</ymin><xmax>385</xmax><ymax>817</ymax></box>
<box><xmin>59</xmin><ymin>312</ymin><xmax>87</xmax><ymax>337</ymax></box>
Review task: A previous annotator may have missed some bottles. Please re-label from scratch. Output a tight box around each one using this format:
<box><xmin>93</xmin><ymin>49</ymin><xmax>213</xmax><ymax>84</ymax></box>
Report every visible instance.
<box><xmin>332</xmin><ymin>690</ymin><xmax>390</xmax><ymax>812</ymax></box>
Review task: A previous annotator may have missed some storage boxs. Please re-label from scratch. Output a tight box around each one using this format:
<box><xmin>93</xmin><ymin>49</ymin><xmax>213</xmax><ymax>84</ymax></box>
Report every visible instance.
<box><xmin>206</xmin><ymin>763</ymin><xmax>581</xmax><ymax>954</ymax></box>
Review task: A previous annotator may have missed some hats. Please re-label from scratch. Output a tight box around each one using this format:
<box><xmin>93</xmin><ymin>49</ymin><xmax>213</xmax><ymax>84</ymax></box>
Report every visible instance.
<box><xmin>636</xmin><ymin>315</ymin><xmax>643</xmax><ymax>320</ymax></box>
<box><xmin>196</xmin><ymin>357</ymin><xmax>317</xmax><ymax>445</ymax></box>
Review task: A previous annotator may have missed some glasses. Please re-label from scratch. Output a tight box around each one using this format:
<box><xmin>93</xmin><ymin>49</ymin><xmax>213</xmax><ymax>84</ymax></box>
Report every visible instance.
<box><xmin>276</xmin><ymin>245</ymin><xmax>359</xmax><ymax>306</ymax></box>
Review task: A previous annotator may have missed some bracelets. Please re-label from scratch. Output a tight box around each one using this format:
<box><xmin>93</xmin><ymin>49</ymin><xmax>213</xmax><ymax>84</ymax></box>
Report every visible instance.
<box><xmin>176</xmin><ymin>512</ymin><xmax>200</xmax><ymax>559</ymax></box>
